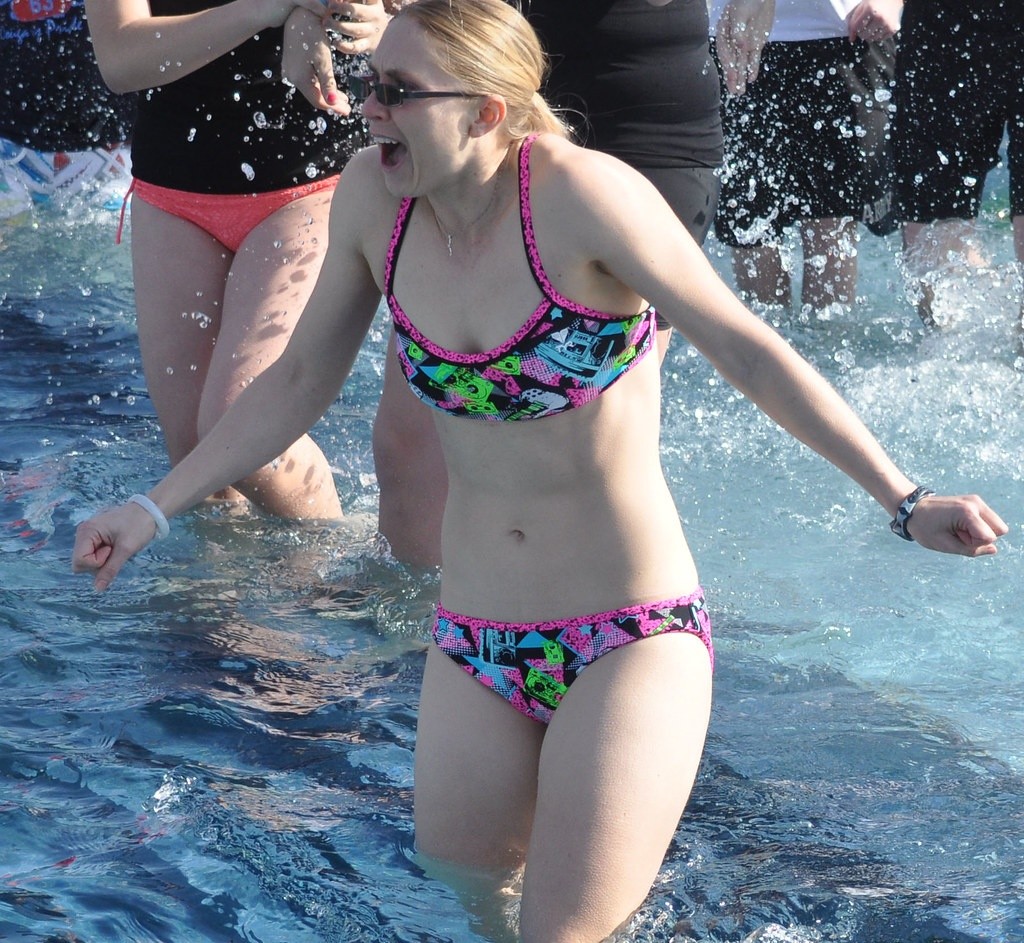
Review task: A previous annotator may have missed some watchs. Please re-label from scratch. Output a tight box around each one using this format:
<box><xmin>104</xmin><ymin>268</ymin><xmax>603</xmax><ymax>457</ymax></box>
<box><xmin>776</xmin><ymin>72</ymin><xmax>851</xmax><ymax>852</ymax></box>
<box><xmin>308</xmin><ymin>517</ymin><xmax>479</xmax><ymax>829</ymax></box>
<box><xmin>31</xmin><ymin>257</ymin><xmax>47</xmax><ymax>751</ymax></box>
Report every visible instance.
<box><xmin>888</xmin><ymin>483</ymin><xmax>937</xmax><ymax>542</ymax></box>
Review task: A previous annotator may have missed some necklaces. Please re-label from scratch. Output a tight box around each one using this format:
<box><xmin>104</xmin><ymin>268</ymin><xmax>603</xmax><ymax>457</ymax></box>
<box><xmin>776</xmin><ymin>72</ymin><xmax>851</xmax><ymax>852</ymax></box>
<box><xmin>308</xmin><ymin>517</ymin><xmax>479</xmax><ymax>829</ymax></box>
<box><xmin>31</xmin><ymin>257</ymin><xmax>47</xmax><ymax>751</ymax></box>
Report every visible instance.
<box><xmin>434</xmin><ymin>176</ymin><xmax>500</xmax><ymax>256</ymax></box>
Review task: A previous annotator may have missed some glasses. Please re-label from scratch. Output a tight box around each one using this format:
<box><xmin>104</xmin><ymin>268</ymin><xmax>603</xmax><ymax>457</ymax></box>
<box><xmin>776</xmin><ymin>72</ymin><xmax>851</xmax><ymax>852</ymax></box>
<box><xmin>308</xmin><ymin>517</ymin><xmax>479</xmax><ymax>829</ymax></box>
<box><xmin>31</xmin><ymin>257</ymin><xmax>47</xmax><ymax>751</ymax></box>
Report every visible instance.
<box><xmin>348</xmin><ymin>74</ymin><xmax>485</xmax><ymax>108</ymax></box>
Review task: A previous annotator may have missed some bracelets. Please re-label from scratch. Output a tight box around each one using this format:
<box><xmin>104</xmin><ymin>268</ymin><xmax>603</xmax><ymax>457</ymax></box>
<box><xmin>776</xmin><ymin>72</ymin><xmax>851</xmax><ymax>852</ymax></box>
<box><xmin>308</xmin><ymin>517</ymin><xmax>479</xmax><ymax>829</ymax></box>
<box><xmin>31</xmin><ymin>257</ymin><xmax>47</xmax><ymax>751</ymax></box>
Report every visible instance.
<box><xmin>126</xmin><ymin>494</ymin><xmax>170</xmax><ymax>540</ymax></box>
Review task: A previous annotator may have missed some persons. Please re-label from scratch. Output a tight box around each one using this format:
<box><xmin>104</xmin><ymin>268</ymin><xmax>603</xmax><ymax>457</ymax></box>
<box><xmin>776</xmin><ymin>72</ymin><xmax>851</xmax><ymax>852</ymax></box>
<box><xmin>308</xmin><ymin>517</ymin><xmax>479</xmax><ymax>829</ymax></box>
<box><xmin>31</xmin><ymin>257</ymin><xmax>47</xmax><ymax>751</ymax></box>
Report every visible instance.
<box><xmin>714</xmin><ymin>0</ymin><xmax>1023</xmax><ymax>359</ymax></box>
<box><xmin>280</xmin><ymin>0</ymin><xmax>723</xmax><ymax>579</ymax></box>
<box><xmin>79</xmin><ymin>0</ymin><xmax>398</xmax><ymax>523</ymax></box>
<box><xmin>704</xmin><ymin>0</ymin><xmax>907</xmax><ymax>313</ymax></box>
<box><xmin>72</xmin><ymin>0</ymin><xmax>1011</xmax><ymax>943</ymax></box>
<box><xmin>0</xmin><ymin>0</ymin><xmax>133</xmax><ymax>227</ymax></box>
